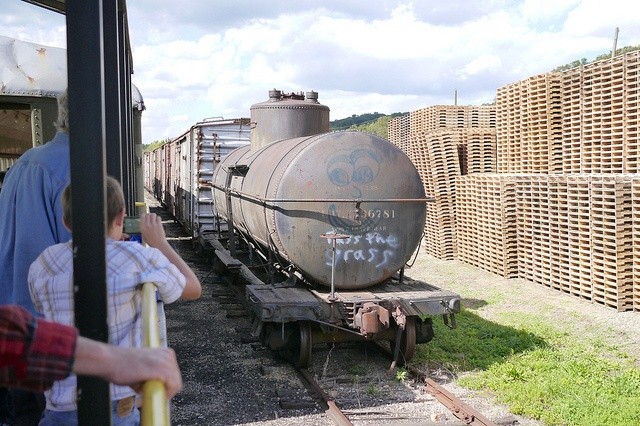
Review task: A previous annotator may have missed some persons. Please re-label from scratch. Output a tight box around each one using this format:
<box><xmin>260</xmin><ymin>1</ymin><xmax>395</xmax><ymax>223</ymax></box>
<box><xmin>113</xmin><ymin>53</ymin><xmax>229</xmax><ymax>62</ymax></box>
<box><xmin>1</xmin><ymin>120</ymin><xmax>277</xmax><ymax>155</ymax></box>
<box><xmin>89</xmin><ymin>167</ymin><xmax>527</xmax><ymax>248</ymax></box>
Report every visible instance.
<box><xmin>0</xmin><ymin>88</ymin><xmax>72</xmax><ymax>426</ymax></box>
<box><xmin>1</xmin><ymin>304</ymin><xmax>182</xmax><ymax>399</ymax></box>
<box><xmin>26</xmin><ymin>175</ymin><xmax>201</xmax><ymax>426</ymax></box>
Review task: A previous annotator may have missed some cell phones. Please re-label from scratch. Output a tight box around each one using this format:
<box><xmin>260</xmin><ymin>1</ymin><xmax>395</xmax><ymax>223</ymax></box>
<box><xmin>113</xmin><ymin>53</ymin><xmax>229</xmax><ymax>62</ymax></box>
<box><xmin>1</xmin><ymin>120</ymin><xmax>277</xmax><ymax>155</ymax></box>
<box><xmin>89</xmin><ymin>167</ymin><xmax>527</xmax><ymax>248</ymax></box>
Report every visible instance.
<box><xmin>122</xmin><ymin>215</ymin><xmax>143</xmax><ymax>236</ymax></box>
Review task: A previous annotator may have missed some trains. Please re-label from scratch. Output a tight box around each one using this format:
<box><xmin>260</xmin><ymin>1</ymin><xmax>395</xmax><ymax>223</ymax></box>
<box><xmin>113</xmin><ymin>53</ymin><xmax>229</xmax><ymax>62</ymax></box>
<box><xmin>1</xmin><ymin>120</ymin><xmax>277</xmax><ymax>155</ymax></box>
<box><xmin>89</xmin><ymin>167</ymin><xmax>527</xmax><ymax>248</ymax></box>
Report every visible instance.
<box><xmin>143</xmin><ymin>89</ymin><xmax>460</xmax><ymax>369</ymax></box>
